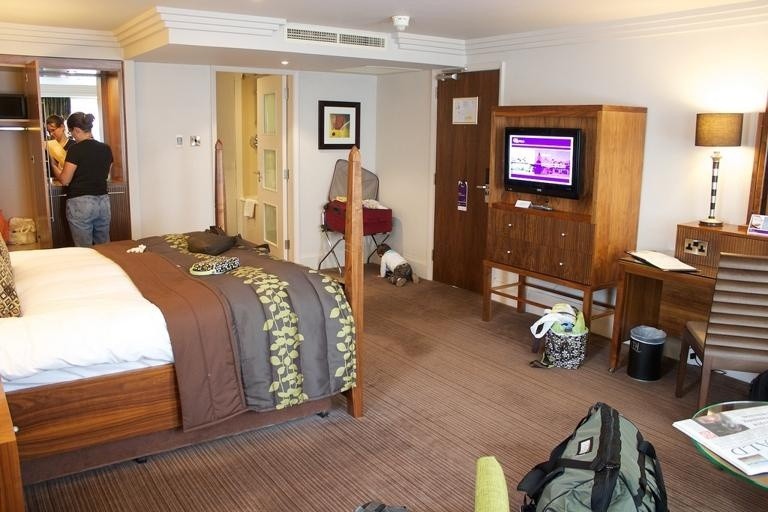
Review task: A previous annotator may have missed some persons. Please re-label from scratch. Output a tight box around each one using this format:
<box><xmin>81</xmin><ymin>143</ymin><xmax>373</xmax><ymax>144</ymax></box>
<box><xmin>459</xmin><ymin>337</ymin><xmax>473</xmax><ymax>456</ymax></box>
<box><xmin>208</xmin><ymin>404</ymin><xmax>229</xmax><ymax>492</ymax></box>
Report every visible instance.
<box><xmin>375</xmin><ymin>242</ymin><xmax>419</xmax><ymax>287</ymax></box>
<box><xmin>41</xmin><ymin>115</ymin><xmax>77</xmax><ymax>179</ymax></box>
<box><xmin>49</xmin><ymin>110</ymin><xmax>116</xmax><ymax>248</ymax></box>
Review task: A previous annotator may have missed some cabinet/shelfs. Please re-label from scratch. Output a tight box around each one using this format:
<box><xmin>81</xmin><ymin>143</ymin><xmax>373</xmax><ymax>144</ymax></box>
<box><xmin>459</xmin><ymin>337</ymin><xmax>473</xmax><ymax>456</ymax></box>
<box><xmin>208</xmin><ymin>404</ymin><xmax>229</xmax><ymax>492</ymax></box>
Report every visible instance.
<box><xmin>318</xmin><ymin>225</ymin><xmax>392</xmax><ymax>279</ymax></box>
<box><xmin>676</xmin><ymin>217</ymin><xmax>768</xmax><ymax>266</ymax></box>
<box><xmin>479</xmin><ymin>103</ymin><xmax>647</xmax><ymax>359</ymax></box>
<box><xmin>0</xmin><ymin>59</ymin><xmax>52</xmax><ymax>251</ymax></box>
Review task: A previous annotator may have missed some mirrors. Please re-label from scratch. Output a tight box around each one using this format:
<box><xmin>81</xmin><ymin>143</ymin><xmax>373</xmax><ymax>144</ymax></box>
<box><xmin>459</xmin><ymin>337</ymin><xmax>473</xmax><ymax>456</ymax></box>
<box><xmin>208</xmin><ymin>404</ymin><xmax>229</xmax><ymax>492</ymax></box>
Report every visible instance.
<box><xmin>39</xmin><ymin>66</ymin><xmax>105</xmax><ymax>182</ymax></box>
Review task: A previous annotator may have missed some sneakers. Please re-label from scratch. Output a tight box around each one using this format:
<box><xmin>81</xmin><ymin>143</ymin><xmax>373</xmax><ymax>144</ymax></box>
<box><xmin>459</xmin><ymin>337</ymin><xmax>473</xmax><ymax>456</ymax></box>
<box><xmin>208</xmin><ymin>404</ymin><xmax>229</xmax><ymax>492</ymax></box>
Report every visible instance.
<box><xmin>395</xmin><ymin>277</ymin><xmax>407</xmax><ymax>287</ymax></box>
<box><xmin>411</xmin><ymin>273</ymin><xmax>419</xmax><ymax>284</ymax></box>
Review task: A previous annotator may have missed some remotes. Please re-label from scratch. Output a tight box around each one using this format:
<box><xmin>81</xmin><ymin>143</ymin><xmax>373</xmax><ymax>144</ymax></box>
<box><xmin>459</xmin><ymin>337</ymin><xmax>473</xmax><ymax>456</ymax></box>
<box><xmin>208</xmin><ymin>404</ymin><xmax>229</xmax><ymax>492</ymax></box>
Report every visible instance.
<box><xmin>530</xmin><ymin>203</ymin><xmax>553</xmax><ymax>211</ymax></box>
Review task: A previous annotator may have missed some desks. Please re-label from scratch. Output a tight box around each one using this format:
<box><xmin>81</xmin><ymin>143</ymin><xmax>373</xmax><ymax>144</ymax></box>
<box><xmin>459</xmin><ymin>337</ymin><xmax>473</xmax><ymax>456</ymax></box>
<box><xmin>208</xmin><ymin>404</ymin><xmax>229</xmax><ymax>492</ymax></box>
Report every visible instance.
<box><xmin>691</xmin><ymin>400</ymin><xmax>768</xmax><ymax>492</ymax></box>
<box><xmin>609</xmin><ymin>255</ymin><xmax>718</xmax><ymax>374</ymax></box>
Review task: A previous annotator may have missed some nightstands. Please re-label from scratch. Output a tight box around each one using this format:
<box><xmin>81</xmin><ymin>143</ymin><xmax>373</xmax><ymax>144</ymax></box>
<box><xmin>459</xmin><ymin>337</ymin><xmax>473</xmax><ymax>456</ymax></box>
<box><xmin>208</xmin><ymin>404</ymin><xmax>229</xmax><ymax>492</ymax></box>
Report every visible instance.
<box><xmin>0</xmin><ymin>377</ymin><xmax>26</xmax><ymax>512</ymax></box>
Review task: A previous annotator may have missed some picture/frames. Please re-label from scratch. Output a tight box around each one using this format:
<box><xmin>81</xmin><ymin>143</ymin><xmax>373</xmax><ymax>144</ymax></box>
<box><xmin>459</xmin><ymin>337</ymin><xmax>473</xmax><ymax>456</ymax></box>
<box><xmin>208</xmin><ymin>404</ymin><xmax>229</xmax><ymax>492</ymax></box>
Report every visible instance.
<box><xmin>317</xmin><ymin>100</ymin><xmax>361</xmax><ymax>149</ymax></box>
<box><xmin>746</xmin><ymin>214</ymin><xmax>768</xmax><ymax>236</ymax></box>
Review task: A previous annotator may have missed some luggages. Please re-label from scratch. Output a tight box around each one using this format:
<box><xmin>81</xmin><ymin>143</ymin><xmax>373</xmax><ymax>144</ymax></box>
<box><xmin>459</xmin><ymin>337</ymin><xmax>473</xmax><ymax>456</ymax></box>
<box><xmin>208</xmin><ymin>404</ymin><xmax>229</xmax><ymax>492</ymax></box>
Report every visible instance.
<box><xmin>323</xmin><ymin>159</ymin><xmax>392</xmax><ymax>235</ymax></box>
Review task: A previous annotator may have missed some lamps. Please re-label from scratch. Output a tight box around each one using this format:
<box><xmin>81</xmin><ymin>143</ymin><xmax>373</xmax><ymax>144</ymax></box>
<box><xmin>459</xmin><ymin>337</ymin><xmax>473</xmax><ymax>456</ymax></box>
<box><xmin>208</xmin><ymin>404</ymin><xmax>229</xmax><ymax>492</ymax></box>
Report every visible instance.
<box><xmin>390</xmin><ymin>16</ymin><xmax>410</xmax><ymax>34</ymax></box>
<box><xmin>695</xmin><ymin>111</ymin><xmax>743</xmax><ymax>228</ymax></box>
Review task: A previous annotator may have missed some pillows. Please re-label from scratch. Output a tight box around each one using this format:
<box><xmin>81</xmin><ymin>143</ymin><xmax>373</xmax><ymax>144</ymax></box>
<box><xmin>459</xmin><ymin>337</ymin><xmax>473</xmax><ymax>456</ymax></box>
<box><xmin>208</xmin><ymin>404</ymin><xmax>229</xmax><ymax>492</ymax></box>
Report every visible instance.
<box><xmin>187</xmin><ymin>232</ymin><xmax>237</xmax><ymax>255</ymax></box>
<box><xmin>0</xmin><ymin>232</ymin><xmax>14</xmax><ymax>275</ymax></box>
<box><xmin>0</xmin><ymin>253</ymin><xmax>23</xmax><ymax>319</ymax></box>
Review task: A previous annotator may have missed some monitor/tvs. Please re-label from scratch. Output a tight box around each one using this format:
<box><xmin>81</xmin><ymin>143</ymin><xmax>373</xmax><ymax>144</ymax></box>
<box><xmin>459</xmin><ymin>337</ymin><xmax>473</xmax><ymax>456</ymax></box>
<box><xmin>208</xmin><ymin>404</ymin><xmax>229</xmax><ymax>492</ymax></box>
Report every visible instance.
<box><xmin>504</xmin><ymin>127</ymin><xmax>580</xmax><ymax>201</ymax></box>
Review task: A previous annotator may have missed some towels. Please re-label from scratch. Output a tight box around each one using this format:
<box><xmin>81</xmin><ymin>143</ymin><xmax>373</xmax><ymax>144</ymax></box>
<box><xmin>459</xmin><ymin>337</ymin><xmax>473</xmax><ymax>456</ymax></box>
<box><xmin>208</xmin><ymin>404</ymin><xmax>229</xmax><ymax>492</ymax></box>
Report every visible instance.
<box><xmin>243</xmin><ymin>199</ymin><xmax>256</xmax><ymax>217</ymax></box>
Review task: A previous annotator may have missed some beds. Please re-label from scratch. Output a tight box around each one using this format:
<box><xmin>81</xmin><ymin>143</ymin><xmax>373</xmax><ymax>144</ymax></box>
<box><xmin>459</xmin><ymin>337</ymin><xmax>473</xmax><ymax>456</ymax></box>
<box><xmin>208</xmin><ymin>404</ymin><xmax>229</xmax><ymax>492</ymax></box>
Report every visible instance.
<box><xmin>0</xmin><ymin>137</ymin><xmax>364</xmax><ymax>485</ymax></box>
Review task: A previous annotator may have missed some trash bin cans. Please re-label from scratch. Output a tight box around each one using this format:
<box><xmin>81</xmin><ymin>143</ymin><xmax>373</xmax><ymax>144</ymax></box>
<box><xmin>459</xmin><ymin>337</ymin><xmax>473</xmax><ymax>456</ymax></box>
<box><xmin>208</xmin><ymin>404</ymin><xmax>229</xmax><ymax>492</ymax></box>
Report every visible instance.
<box><xmin>626</xmin><ymin>325</ymin><xmax>667</xmax><ymax>382</ymax></box>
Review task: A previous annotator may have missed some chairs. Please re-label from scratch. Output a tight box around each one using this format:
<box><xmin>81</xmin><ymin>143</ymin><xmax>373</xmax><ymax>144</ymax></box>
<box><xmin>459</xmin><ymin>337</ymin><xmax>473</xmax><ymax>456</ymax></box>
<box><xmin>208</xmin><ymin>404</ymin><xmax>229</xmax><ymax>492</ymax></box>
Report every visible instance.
<box><xmin>674</xmin><ymin>251</ymin><xmax>768</xmax><ymax>411</ymax></box>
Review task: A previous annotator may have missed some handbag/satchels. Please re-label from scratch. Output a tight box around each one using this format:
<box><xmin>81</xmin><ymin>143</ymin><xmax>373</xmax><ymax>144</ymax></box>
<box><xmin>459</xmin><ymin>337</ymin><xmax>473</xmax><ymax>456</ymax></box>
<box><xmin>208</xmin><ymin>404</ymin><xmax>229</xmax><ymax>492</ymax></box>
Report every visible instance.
<box><xmin>187</xmin><ymin>226</ymin><xmax>271</xmax><ymax>256</ymax></box>
<box><xmin>517</xmin><ymin>401</ymin><xmax>670</xmax><ymax>511</ymax></box>
<box><xmin>529</xmin><ymin>303</ymin><xmax>589</xmax><ymax>371</ymax></box>
